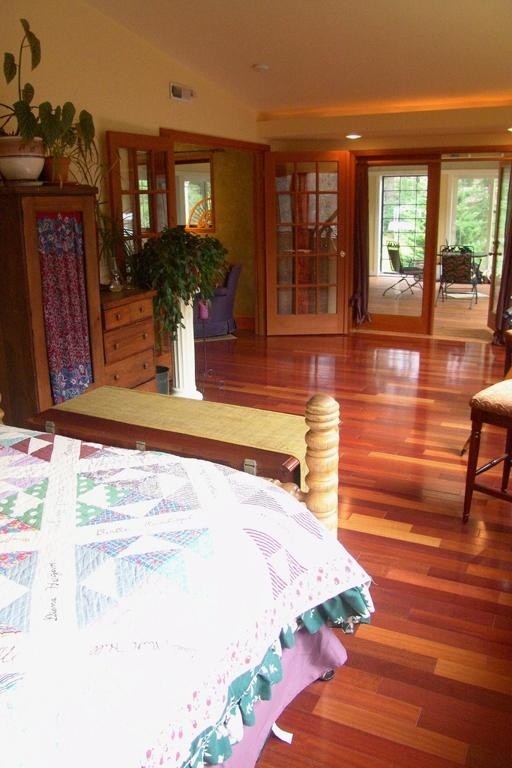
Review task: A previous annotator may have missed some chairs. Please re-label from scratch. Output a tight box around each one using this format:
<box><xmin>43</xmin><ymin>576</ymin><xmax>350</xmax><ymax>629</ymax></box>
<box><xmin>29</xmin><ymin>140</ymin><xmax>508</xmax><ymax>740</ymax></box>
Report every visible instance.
<box><xmin>383</xmin><ymin>245</ymin><xmax>481</xmax><ymax>309</ymax></box>
<box><xmin>194</xmin><ymin>264</ymin><xmax>240</xmax><ymax>338</ymax></box>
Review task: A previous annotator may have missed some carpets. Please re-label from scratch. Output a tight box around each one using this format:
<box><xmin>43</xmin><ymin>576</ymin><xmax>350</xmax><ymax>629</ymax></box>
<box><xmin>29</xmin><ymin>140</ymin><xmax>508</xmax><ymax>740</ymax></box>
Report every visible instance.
<box><xmin>194</xmin><ymin>334</ymin><xmax>239</xmax><ymax>342</ymax></box>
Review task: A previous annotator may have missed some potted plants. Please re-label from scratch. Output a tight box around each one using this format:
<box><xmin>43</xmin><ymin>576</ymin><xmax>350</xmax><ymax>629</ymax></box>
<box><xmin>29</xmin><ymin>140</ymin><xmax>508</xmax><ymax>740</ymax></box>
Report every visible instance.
<box><xmin>0</xmin><ymin>19</ymin><xmax>95</xmax><ymax>186</ymax></box>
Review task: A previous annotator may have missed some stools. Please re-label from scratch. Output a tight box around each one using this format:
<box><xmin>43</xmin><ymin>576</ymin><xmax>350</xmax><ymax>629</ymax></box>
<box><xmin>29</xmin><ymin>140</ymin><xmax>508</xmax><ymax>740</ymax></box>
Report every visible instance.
<box><xmin>503</xmin><ymin>329</ymin><xmax>512</xmax><ymax>377</ymax></box>
<box><xmin>462</xmin><ymin>378</ymin><xmax>512</xmax><ymax>523</ymax></box>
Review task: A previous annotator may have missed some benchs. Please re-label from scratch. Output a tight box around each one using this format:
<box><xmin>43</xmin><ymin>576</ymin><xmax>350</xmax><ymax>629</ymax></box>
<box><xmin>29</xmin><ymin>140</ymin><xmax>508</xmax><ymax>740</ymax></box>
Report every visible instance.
<box><xmin>28</xmin><ymin>385</ymin><xmax>309</xmax><ymax>483</ymax></box>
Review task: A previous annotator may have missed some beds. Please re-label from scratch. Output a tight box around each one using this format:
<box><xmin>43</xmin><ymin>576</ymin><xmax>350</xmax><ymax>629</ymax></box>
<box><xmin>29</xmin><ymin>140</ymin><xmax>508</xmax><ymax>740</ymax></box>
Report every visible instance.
<box><xmin>0</xmin><ymin>393</ymin><xmax>376</xmax><ymax>768</ymax></box>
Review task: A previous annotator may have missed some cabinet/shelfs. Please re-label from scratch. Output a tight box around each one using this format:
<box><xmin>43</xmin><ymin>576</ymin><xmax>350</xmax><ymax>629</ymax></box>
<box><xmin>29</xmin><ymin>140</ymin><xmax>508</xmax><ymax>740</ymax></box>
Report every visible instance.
<box><xmin>0</xmin><ymin>184</ymin><xmax>157</xmax><ymax>423</ymax></box>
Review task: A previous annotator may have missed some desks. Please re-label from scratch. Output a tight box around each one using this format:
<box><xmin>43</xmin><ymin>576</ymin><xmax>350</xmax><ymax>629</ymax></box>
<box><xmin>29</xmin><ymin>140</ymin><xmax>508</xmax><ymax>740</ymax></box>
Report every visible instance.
<box><xmin>436</xmin><ymin>252</ymin><xmax>487</xmax><ymax>299</ymax></box>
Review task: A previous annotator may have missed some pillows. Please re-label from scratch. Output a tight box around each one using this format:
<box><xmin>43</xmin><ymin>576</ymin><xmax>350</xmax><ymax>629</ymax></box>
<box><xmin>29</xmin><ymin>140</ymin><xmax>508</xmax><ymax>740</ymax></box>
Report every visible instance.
<box><xmin>210</xmin><ymin>287</ymin><xmax>227</xmax><ymax>296</ymax></box>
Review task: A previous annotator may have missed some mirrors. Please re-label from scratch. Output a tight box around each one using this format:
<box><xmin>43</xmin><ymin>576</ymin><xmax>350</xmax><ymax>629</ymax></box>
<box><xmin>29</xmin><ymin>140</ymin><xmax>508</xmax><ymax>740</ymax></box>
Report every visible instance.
<box><xmin>134</xmin><ymin>158</ymin><xmax>216</xmax><ymax>234</ymax></box>
